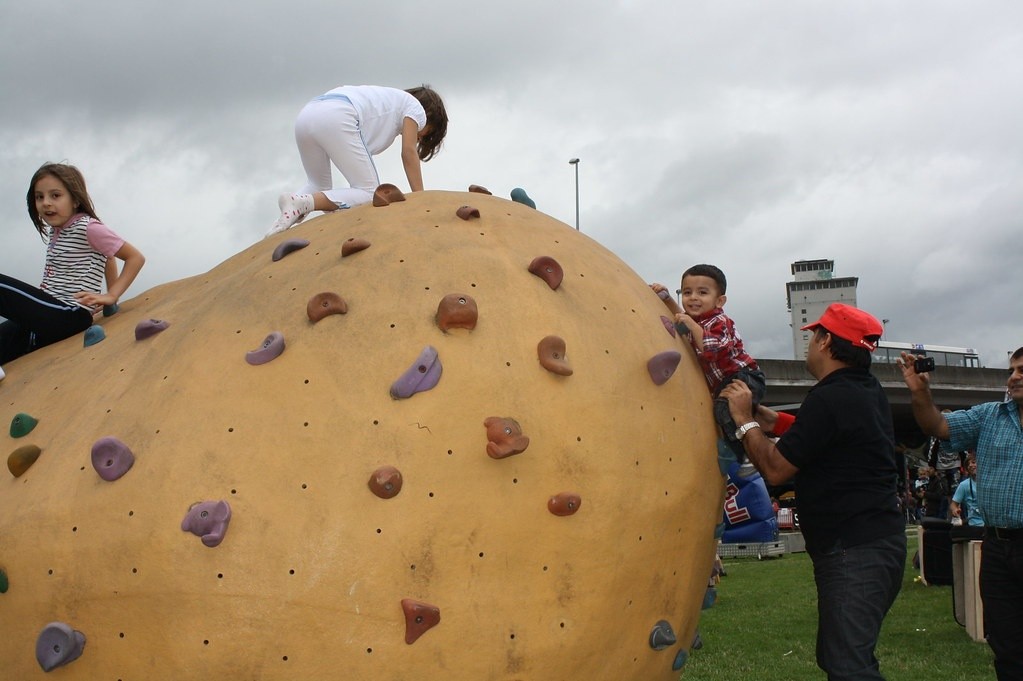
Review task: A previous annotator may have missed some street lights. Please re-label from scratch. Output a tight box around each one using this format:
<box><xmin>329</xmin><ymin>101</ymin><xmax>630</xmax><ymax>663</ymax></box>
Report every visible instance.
<box><xmin>569</xmin><ymin>158</ymin><xmax>580</xmax><ymax>232</ymax></box>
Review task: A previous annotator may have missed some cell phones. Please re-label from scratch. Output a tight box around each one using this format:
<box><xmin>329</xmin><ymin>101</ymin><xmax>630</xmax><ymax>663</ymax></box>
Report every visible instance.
<box><xmin>913</xmin><ymin>357</ymin><xmax>935</xmax><ymax>374</ymax></box>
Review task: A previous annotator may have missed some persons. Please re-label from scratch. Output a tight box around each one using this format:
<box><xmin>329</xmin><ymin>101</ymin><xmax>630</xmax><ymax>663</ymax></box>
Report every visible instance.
<box><xmin>267</xmin><ymin>85</ymin><xmax>449</xmax><ymax>237</ymax></box>
<box><xmin>896</xmin><ymin>408</ymin><xmax>984</xmax><ymax>526</ymax></box>
<box><xmin>721</xmin><ymin>304</ymin><xmax>908</xmax><ymax>681</ymax></box>
<box><xmin>0</xmin><ymin>159</ymin><xmax>145</xmax><ymax>381</ymax></box>
<box><xmin>651</xmin><ymin>264</ymin><xmax>765</xmax><ymax>480</ymax></box>
<box><xmin>896</xmin><ymin>347</ymin><xmax>1023</xmax><ymax>681</ymax></box>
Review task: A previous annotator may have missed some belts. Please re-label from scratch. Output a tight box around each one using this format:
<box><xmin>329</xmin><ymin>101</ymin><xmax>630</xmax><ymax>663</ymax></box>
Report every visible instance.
<box><xmin>986</xmin><ymin>526</ymin><xmax>1023</xmax><ymax>540</ymax></box>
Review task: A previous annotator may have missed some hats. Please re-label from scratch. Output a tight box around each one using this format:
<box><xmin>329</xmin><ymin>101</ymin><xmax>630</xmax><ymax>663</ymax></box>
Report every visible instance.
<box><xmin>799</xmin><ymin>303</ymin><xmax>883</xmax><ymax>352</ymax></box>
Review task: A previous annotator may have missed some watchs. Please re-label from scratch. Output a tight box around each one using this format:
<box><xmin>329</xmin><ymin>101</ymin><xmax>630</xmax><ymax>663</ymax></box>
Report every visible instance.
<box><xmin>735</xmin><ymin>422</ymin><xmax>760</xmax><ymax>442</ymax></box>
